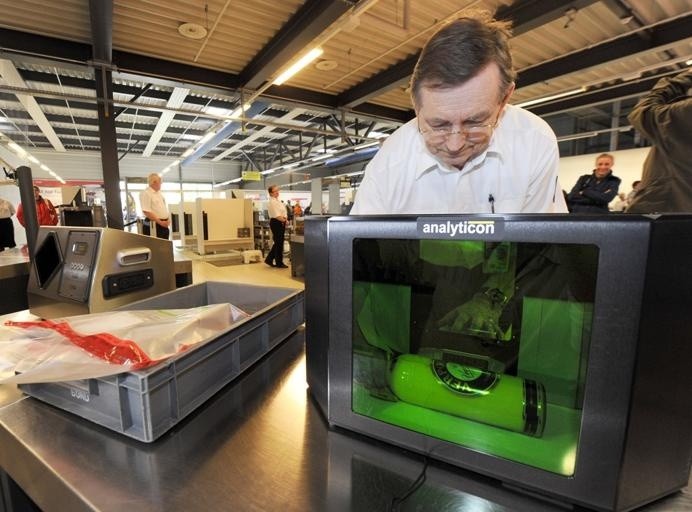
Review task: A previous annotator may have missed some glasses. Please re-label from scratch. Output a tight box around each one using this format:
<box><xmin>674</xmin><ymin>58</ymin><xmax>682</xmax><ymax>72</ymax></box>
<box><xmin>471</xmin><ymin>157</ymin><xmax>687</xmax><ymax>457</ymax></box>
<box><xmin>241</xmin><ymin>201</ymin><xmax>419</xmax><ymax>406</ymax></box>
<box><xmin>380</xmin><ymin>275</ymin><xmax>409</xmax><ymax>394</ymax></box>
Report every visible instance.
<box><xmin>417</xmin><ymin>105</ymin><xmax>502</xmax><ymax>138</ymax></box>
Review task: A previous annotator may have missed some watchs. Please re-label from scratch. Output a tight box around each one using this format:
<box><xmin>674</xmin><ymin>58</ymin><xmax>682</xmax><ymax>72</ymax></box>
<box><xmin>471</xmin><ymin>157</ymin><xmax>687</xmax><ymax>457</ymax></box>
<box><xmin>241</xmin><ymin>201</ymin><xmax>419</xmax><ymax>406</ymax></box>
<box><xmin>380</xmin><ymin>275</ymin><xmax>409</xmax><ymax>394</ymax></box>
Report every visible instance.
<box><xmin>480</xmin><ymin>286</ymin><xmax>508</xmax><ymax>308</ymax></box>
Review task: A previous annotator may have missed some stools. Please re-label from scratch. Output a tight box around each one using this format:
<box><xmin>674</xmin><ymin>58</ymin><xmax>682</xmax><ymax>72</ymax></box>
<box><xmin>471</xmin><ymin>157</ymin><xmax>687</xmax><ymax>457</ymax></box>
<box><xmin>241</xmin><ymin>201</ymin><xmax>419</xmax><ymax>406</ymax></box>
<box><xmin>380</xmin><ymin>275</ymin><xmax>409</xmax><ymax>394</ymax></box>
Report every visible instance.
<box><xmin>241</xmin><ymin>249</ymin><xmax>262</xmax><ymax>264</ymax></box>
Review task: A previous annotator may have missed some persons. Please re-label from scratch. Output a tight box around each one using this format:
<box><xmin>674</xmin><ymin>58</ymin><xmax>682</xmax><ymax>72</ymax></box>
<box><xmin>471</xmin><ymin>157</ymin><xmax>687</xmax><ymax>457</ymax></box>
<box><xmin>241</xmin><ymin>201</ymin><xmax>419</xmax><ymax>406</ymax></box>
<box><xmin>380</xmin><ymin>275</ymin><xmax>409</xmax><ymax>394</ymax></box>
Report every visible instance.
<box><xmin>282</xmin><ymin>200</ymin><xmax>354</xmax><ymax>219</ymax></box>
<box><xmin>0</xmin><ymin>198</ymin><xmax>16</xmax><ymax>252</ymax></box>
<box><xmin>624</xmin><ymin>69</ymin><xmax>692</xmax><ymax>213</ymax></box>
<box><xmin>16</xmin><ymin>186</ymin><xmax>58</xmax><ymax>228</ymax></box>
<box><xmin>264</xmin><ymin>185</ymin><xmax>288</xmax><ymax>268</ymax></box>
<box><xmin>141</xmin><ymin>173</ymin><xmax>171</xmax><ymax>240</ymax></box>
<box><xmin>349</xmin><ymin>8</ymin><xmax>573</xmax><ymax>390</ymax></box>
<box><xmin>626</xmin><ymin>181</ymin><xmax>641</xmax><ymax>204</ymax></box>
<box><xmin>566</xmin><ymin>154</ymin><xmax>621</xmax><ymax>212</ymax></box>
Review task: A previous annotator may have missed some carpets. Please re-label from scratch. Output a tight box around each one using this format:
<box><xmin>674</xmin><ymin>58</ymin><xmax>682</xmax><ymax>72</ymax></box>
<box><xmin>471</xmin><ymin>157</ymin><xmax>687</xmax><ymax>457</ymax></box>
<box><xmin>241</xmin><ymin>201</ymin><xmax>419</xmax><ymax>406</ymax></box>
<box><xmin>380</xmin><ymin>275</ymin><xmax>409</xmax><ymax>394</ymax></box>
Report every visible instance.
<box><xmin>206</xmin><ymin>256</ymin><xmax>261</xmax><ymax>268</ymax></box>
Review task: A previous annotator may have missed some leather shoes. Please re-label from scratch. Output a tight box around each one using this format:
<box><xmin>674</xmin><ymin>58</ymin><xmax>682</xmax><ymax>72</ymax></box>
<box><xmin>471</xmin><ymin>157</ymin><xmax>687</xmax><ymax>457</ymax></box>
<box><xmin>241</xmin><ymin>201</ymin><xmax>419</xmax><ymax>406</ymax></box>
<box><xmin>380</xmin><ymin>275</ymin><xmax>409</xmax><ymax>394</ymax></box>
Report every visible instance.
<box><xmin>264</xmin><ymin>259</ymin><xmax>288</xmax><ymax>268</ymax></box>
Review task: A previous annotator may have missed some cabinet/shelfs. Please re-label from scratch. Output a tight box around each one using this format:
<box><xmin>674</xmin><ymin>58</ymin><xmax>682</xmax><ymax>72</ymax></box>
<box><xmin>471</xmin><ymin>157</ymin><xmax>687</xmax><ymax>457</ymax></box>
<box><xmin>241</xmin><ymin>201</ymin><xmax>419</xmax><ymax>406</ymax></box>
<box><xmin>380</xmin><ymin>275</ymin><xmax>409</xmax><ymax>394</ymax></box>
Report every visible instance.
<box><xmin>252</xmin><ymin>223</ymin><xmax>292</xmax><ymax>258</ymax></box>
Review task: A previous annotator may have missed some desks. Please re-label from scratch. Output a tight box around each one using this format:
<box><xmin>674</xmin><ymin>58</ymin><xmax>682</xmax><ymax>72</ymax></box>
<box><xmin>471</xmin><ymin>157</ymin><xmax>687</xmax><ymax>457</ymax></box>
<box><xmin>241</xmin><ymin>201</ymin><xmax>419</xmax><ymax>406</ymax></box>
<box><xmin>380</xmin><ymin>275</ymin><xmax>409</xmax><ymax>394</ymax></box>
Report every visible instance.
<box><xmin>0</xmin><ymin>300</ymin><xmax>691</xmax><ymax>510</ymax></box>
